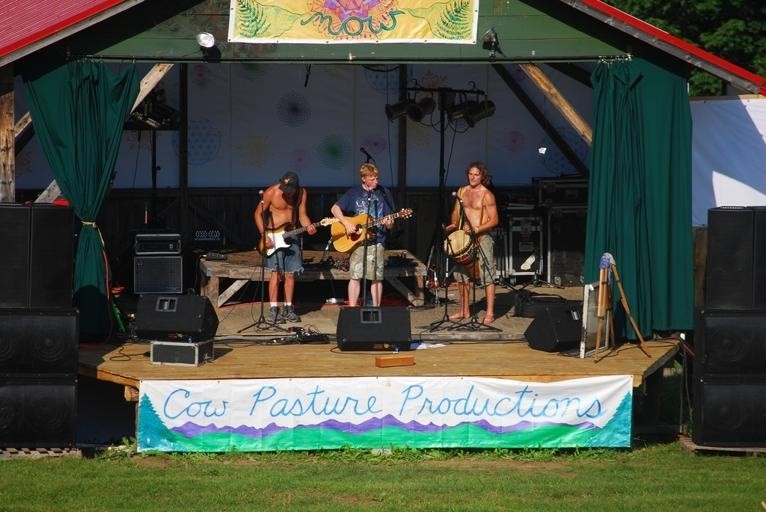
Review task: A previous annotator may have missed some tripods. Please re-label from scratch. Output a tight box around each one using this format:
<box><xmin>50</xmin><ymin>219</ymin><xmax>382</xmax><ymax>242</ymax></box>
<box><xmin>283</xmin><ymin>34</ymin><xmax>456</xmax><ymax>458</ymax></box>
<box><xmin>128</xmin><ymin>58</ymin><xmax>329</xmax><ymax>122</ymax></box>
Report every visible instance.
<box><xmin>237</xmin><ymin>204</ymin><xmax>289</xmax><ymax>334</ymax></box>
<box><xmin>430</xmin><ymin>238</ymin><xmax>474</xmax><ymax>332</ymax></box>
<box><xmin>448</xmin><ymin>236</ymin><xmax>504</xmax><ymax>332</ymax></box>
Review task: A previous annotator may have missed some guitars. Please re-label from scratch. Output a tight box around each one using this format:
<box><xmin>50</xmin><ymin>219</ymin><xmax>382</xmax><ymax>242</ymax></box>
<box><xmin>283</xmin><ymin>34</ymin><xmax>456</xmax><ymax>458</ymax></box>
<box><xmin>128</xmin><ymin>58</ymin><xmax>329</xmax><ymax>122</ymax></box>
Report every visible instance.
<box><xmin>257</xmin><ymin>216</ymin><xmax>340</xmax><ymax>259</ymax></box>
<box><xmin>331</xmin><ymin>208</ymin><xmax>413</xmax><ymax>253</ymax></box>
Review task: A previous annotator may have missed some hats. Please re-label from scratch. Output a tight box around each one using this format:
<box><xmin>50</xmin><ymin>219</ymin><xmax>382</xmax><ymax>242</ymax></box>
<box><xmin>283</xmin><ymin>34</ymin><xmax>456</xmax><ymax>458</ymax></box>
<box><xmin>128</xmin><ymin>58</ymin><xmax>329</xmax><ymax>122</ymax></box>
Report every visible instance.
<box><xmin>279</xmin><ymin>172</ymin><xmax>299</xmax><ymax>192</ymax></box>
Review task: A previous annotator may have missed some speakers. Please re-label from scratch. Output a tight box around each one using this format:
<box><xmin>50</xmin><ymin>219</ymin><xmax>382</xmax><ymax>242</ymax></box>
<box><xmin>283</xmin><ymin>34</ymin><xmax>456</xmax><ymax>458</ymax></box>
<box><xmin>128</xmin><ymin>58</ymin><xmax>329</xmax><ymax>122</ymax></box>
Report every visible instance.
<box><xmin>336</xmin><ymin>305</ymin><xmax>413</xmax><ymax>351</ymax></box>
<box><xmin>133</xmin><ymin>293</ymin><xmax>220</xmax><ymax>340</ymax></box>
<box><xmin>0</xmin><ymin>202</ymin><xmax>75</xmax><ymax>309</ymax></box>
<box><xmin>691</xmin><ymin>308</ymin><xmax>765</xmax><ymax>448</ymax></box>
<box><xmin>524</xmin><ymin>304</ymin><xmax>582</xmax><ymax>353</ymax></box>
<box><xmin>701</xmin><ymin>204</ymin><xmax>765</xmax><ymax>312</ymax></box>
<box><xmin>0</xmin><ymin>307</ymin><xmax>81</xmax><ymax>448</ymax></box>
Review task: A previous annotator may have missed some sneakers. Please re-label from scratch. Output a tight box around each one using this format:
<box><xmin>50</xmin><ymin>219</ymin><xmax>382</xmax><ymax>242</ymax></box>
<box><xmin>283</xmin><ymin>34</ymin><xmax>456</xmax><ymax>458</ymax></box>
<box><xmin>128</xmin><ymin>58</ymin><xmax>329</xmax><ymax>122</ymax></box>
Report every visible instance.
<box><xmin>281</xmin><ymin>304</ymin><xmax>301</xmax><ymax>322</ymax></box>
<box><xmin>268</xmin><ymin>305</ymin><xmax>286</xmax><ymax>324</ymax></box>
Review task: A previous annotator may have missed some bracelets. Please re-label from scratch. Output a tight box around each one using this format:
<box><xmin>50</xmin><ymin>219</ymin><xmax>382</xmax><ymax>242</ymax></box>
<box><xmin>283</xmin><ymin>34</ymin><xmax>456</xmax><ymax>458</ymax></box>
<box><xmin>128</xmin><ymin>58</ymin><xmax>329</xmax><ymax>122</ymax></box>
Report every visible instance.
<box><xmin>475</xmin><ymin>226</ymin><xmax>479</xmax><ymax>235</ymax></box>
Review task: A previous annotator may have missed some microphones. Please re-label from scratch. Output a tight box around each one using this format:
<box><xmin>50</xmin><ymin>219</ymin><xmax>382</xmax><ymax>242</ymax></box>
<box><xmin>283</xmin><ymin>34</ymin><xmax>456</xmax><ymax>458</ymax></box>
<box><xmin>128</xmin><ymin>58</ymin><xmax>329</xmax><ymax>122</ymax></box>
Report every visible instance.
<box><xmin>259</xmin><ymin>190</ymin><xmax>264</xmax><ymax>204</ymax></box>
<box><xmin>360</xmin><ymin>147</ymin><xmax>375</xmax><ymax>162</ymax></box>
<box><xmin>452</xmin><ymin>192</ymin><xmax>463</xmax><ymax>203</ymax></box>
<box><xmin>366</xmin><ymin>187</ymin><xmax>373</xmax><ymax>202</ymax></box>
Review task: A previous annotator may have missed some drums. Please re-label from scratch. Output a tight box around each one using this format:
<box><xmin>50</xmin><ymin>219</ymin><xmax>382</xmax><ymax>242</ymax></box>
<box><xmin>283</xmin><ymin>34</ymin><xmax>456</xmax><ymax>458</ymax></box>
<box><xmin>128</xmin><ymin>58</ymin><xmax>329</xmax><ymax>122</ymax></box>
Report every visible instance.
<box><xmin>444</xmin><ymin>229</ymin><xmax>484</xmax><ymax>289</ymax></box>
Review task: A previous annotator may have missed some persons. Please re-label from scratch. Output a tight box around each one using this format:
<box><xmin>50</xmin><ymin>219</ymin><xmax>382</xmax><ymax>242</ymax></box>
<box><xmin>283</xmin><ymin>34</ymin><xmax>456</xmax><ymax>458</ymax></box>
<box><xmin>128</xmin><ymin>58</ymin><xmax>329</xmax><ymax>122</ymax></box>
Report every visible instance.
<box><xmin>330</xmin><ymin>163</ymin><xmax>398</xmax><ymax>306</ymax></box>
<box><xmin>439</xmin><ymin>160</ymin><xmax>500</xmax><ymax>328</ymax></box>
<box><xmin>252</xmin><ymin>170</ymin><xmax>317</xmax><ymax>326</ymax></box>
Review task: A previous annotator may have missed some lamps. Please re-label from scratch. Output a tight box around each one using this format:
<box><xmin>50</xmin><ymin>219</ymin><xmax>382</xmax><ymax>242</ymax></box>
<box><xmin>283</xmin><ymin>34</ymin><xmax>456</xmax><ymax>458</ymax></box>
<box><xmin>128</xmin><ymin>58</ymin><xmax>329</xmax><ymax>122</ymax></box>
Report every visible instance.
<box><xmin>386</xmin><ymin>80</ymin><xmax>497</xmax><ymax>281</ymax></box>
<box><xmin>482</xmin><ymin>28</ymin><xmax>499</xmax><ymax>55</ymax></box>
<box><xmin>196</xmin><ymin>32</ymin><xmax>217</xmax><ymax>50</ymax></box>
<box><xmin>128</xmin><ymin>98</ymin><xmax>181</xmax><ymax>230</ymax></box>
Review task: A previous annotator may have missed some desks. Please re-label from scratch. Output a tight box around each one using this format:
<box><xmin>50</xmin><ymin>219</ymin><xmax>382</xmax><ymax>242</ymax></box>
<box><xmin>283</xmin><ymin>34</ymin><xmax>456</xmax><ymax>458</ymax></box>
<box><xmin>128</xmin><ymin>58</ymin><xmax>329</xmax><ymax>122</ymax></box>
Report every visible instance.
<box><xmin>194</xmin><ymin>248</ymin><xmax>431</xmax><ymax>308</ymax></box>
